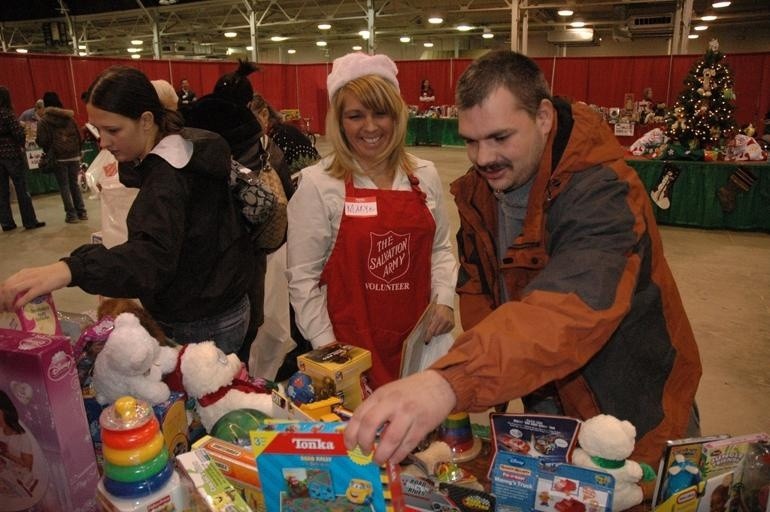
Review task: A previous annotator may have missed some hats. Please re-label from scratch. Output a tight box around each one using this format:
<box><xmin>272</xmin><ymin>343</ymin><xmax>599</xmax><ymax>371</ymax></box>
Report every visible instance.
<box><xmin>327</xmin><ymin>53</ymin><xmax>400</xmax><ymax>103</ymax></box>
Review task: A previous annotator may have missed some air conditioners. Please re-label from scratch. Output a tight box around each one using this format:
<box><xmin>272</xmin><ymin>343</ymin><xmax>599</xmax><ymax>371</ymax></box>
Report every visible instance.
<box><xmin>160</xmin><ymin>42</ymin><xmax>227</xmax><ymax>60</ymax></box>
<box><xmin>547</xmin><ymin>28</ymin><xmax>601</xmax><ymax>47</ymax></box>
<box><xmin>629</xmin><ymin>12</ymin><xmax>674</xmax><ymax>39</ymax></box>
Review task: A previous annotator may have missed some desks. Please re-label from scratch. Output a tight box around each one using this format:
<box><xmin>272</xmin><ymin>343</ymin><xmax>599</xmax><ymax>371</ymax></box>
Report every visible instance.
<box><xmin>619</xmin><ymin>144</ymin><xmax>769</xmax><ymax>236</ymax></box>
<box><xmin>403</xmin><ymin>112</ymin><xmax>468</xmax><ymax>148</ymax></box>
<box><xmin>608</xmin><ymin>123</ymin><xmax>668</xmax><ymax>147</ymax></box>
<box><xmin>20</xmin><ymin>139</ymin><xmax>102</xmax><ymax>196</ymax></box>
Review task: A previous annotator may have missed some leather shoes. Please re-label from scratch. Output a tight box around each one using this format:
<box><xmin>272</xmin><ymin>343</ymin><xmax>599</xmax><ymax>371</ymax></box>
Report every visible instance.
<box><xmin>2</xmin><ymin>222</ymin><xmax>44</xmax><ymax>230</ymax></box>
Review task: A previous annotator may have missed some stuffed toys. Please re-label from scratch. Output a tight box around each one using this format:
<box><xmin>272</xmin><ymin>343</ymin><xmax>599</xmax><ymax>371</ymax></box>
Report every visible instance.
<box><xmin>86</xmin><ymin>297</ymin><xmax>273</xmax><ymax>433</ymax></box>
<box><xmin>571</xmin><ymin>415</ymin><xmax>655</xmax><ymax>511</ymax></box>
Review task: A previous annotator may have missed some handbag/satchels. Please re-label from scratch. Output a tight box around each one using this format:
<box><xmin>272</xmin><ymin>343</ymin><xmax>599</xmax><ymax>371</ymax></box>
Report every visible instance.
<box><xmin>39</xmin><ymin>148</ymin><xmax>59</xmax><ymax>172</ymax></box>
<box><xmin>230</xmin><ymin>152</ymin><xmax>289</xmax><ymax>255</ymax></box>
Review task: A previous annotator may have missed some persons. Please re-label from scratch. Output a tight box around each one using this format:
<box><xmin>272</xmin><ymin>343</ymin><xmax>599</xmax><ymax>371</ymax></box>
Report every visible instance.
<box><xmin>87</xmin><ymin>80</ymin><xmax>178</xmax><ymax>249</ymax></box>
<box><xmin>178</xmin><ymin>72</ymin><xmax>321</xmax><ymax>202</ymax></box>
<box><xmin>0</xmin><ymin>64</ymin><xmax>251</xmax><ymax>353</ymax></box>
<box><xmin>0</xmin><ymin>86</ymin><xmax>88</xmax><ymax>230</ymax></box>
<box><xmin>643</xmin><ymin>87</ymin><xmax>668</xmax><ymax>109</ymax></box>
<box><xmin>418</xmin><ymin>79</ymin><xmax>435</xmax><ymax>110</ymax></box>
<box><xmin>286</xmin><ymin>50</ymin><xmax>459</xmax><ymax>400</ymax></box>
<box><xmin>343</xmin><ymin>50</ymin><xmax>704</xmax><ymax>475</ymax></box>
